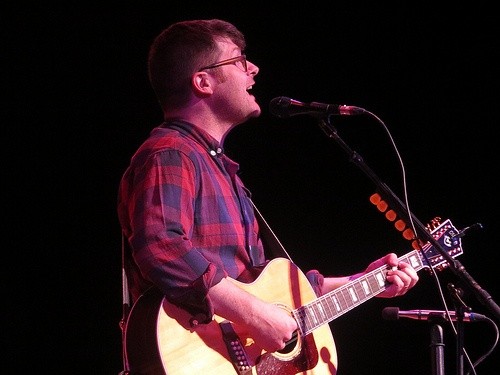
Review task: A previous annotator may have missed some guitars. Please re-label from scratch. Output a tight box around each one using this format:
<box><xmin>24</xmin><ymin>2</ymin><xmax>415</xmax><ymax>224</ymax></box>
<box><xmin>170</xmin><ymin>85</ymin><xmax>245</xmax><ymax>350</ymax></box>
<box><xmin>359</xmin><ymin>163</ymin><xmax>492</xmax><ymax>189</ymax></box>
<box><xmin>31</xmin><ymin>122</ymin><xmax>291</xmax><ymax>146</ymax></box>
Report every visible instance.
<box><xmin>123</xmin><ymin>216</ymin><xmax>484</xmax><ymax>375</ymax></box>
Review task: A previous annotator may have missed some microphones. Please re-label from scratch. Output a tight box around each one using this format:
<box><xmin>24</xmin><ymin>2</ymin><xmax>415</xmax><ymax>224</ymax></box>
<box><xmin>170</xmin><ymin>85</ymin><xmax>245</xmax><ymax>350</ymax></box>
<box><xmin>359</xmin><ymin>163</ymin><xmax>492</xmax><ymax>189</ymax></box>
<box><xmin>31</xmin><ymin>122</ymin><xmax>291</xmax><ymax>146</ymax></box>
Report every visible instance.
<box><xmin>269</xmin><ymin>96</ymin><xmax>365</xmax><ymax>118</ymax></box>
<box><xmin>383</xmin><ymin>307</ymin><xmax>488</xmax><ymax>323</ymax></box>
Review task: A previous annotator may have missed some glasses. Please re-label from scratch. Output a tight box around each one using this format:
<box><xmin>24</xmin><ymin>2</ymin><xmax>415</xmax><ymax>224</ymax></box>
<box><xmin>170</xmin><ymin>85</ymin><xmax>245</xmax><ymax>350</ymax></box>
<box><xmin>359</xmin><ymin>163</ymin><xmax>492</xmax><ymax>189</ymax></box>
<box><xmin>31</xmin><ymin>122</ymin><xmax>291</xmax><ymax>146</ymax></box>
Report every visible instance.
<box><xmin>198</xmin><ymin>54</ymin><xmax>248</xmax><ymax>73</ymax></box>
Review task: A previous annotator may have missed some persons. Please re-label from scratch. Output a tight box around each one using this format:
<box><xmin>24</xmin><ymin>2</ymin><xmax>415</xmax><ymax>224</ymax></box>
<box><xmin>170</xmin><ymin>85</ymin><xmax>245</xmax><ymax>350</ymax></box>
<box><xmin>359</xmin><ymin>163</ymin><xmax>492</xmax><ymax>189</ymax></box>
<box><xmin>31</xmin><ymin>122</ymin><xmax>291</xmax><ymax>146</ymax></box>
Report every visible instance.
<box><xmin>112</xmin><ymin>18</ymin><xmax>419</xmax><ymax>374</ymax></box>
